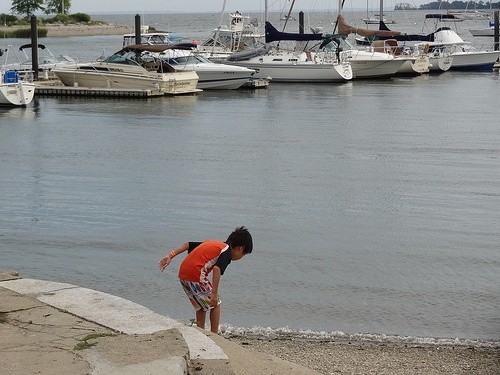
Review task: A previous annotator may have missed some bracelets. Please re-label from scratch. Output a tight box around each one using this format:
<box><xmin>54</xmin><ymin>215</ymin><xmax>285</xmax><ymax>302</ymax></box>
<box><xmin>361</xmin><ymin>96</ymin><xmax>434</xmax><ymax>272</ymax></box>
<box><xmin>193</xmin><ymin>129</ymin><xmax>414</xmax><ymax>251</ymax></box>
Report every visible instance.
<box><xmin>171</xmin><ymin>251</ymin><xmax>176</xmax><ymax>257</ymax></box>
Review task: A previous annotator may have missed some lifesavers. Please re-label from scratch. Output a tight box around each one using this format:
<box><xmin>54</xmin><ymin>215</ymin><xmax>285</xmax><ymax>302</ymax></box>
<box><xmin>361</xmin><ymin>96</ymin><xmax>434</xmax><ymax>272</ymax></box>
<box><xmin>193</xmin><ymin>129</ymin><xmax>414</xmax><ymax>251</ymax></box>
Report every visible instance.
<box><xmin>423</xmin><ymin>43</ymin><xmax>429</xmax><ymax>52</ymax></box>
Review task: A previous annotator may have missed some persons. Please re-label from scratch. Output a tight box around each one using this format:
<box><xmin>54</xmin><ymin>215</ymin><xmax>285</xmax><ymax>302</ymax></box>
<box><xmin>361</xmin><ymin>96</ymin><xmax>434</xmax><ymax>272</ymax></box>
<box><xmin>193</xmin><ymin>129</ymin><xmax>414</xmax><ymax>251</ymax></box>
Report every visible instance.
<box><xmin>158</xmin><ymin>226</ymin><xmax>253</xmax><ymax>334</ymax></box>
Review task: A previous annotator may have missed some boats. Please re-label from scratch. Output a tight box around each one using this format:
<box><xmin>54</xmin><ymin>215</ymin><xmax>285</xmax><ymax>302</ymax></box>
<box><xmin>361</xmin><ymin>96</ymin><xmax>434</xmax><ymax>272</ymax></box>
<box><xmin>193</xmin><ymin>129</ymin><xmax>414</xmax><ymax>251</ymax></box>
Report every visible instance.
<box><xmin>279</xmin><ymin>15</ymin><xmax>296</xmax><ymax>21</ymax></box>
<box><xmin>467</xmin><ymin>28</ymin><xmax>494</xmax><ymax>36</ymax></box>
<box><xmin>440</xmin><ymin>18</ymin><xmax>464</xmax><ymax>22</ymax></box>
<box><xmin>446</xmin><ymin>10</ymin><xmax>491</xmax><ymax>20</ymax></box>
<box><xmin>362</xmin><ymin>14</ymin><xmax>396</xmax><ymax>24</ymax></box>
<box><xmin>0</xmin><ymin>0</ymin><xmax>500</xmax><ymax>91</ymax></box>
